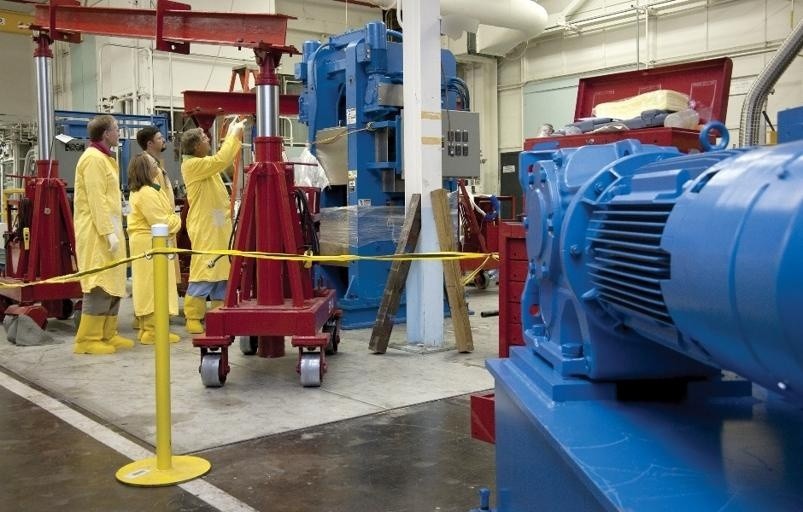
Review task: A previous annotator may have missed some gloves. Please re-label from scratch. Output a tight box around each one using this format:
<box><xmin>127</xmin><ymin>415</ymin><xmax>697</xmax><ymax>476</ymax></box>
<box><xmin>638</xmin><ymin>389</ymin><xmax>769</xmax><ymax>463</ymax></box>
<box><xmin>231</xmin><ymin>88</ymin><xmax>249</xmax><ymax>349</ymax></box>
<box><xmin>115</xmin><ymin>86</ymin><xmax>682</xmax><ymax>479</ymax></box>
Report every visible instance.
<box><xmin>104</xmin><ymin>233</ymin><xmax>120</xmax><ymax>253</ymax></box>
<box><xmin>228</xmin><ymin>114</ymin><xmax>249</xmax><ymax>138</ymax></box>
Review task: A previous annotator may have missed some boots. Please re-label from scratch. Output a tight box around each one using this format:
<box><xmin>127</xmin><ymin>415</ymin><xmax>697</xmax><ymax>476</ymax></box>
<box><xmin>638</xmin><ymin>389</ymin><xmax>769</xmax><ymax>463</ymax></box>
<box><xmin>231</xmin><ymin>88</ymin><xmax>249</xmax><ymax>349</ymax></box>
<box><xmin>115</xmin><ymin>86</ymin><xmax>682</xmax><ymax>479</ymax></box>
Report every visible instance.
<box><xmin>138</xmin><ymin>312</ymin><xmax>181</xmax><ymax>345</ymax></box>
<box><xmin>102</xmin><ymin>316</ymin><xmax>134</xmax><ymax>348</ymax></box>
<box><xmin>184</xmin><ymin>293</ymin><xmax>224</xmax><ymax>334</ymax></box>
<box><xmin>72</xmin><ymin>314</ymin><xmax>116</xmax><ymax>354</ymax></box>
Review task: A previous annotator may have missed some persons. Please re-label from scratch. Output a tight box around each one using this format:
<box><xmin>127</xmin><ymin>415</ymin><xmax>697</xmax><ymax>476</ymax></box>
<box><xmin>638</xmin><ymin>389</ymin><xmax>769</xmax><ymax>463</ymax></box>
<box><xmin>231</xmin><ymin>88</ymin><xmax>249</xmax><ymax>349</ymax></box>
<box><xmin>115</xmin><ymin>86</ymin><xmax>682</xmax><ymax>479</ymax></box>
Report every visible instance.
<box><xmin>70</xmin><ymin>113</ymin><xmax>135</xmax><ymax>356</ymax></box>
<box><xmin>126</xmin><ymin>150</ymin><xmax>181</xmax><ymax>346</ymax></box>
<box><xmin>131</xmin><ymin>125</ymin><xmax>182</xmax><ymax>336</ymax></box>
<box><xmin>179</xmin><ymin>114</ymin><xmax>249</xmax><ymax>335</ymax></box>
